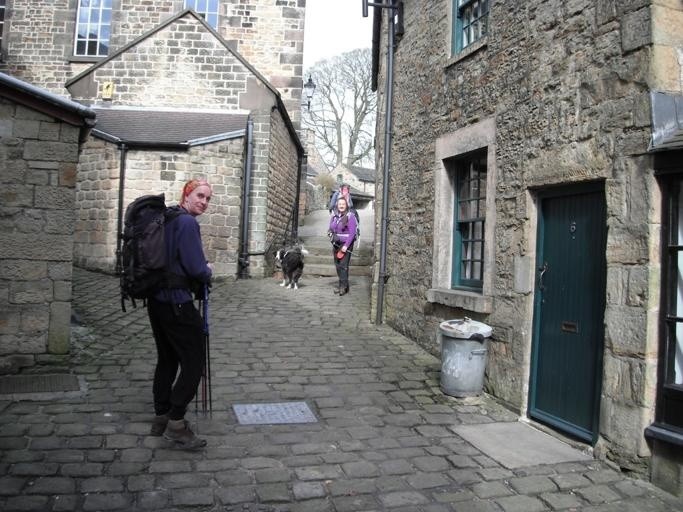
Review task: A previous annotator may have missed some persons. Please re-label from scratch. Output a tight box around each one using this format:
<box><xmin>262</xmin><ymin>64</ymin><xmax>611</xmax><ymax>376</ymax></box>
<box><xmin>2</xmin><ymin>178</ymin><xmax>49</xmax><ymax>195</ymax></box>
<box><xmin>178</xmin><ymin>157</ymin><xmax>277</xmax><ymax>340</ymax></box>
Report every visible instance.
<box><xmin>328</xmin><ymin>183</ymin><xmax>355</xmax><ymax>212</ymax></box>
<box><xmin>145</xmin><ymin>180</ymin><xmax>215</xmax><ymax>451</ymax></box>
<box><xmin>326</xmin><ymin>197</ymin><xmax>357</xmax><ymax>297</ymax></box>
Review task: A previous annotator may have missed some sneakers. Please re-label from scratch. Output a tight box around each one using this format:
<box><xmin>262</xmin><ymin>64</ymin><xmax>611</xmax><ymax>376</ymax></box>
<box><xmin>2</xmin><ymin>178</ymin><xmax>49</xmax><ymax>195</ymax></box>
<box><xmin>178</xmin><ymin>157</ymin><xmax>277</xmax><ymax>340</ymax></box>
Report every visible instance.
<box><xmin>158</xmin><ymin>421</ymin><xmax>207</xmax><ymax>452</ymax></box>
<box><xmin>150</xmin><ymin>414</ymin><xmax>168</xmax><ymax>437</ymax></box>
<box><xmin>333</xmin><ymin>288</ymin><xmax>349</xmax><ymax>296</ymax></box>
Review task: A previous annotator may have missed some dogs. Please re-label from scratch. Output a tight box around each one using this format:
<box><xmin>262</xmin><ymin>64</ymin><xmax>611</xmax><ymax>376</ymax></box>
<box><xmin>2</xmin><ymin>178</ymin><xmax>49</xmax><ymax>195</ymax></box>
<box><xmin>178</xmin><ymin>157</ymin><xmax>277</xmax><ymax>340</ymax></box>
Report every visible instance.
<box><xmin>272</xmin><ymin>249</ymin><xmax>305</xmax><ymax>290</ymax></box>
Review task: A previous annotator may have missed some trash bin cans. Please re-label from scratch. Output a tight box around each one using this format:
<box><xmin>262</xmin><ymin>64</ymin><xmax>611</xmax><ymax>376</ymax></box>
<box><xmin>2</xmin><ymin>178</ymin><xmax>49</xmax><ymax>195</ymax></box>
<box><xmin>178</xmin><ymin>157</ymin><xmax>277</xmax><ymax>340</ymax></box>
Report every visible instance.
<box><xmin>439</xmin><ymin>315</ymin><xmax>494</xmax><ymax>399</ymax></box>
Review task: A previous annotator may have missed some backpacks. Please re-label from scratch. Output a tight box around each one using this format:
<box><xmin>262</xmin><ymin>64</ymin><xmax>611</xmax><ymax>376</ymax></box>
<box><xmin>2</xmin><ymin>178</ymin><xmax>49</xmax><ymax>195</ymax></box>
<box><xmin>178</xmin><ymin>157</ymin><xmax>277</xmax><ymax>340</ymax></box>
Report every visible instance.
<box><xmin>114</xmin><ymin>191</ymin><xmax>203</xmax><ymax>313</ymax></box>
<box><xmin>345</xmin><ymin>212</ymin><xmax>360</xmax><ymax>240</ymax></box>
